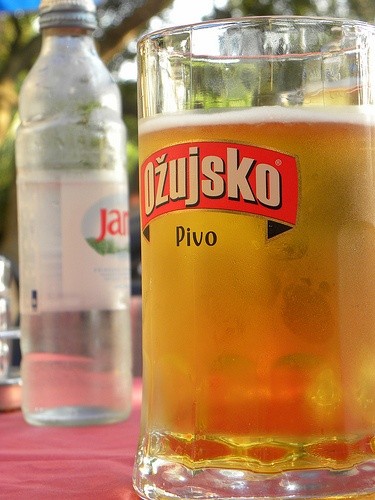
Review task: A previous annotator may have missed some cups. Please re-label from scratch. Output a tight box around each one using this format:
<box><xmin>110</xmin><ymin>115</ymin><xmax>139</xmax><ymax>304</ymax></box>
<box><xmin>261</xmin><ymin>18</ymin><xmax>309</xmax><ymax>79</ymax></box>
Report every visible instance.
<box><xmin>134</xmin><ymin>19</ymin><xmax>375</xmax><ymax>500</ymax></box>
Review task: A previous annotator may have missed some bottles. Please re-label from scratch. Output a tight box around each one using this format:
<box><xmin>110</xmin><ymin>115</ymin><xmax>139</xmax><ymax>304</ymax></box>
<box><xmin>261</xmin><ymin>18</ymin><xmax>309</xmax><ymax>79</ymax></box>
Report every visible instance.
<box><xmin>16</xmin><ymin>0</ymin><xmax>131</xmax><ymax>426</ymax></box>
